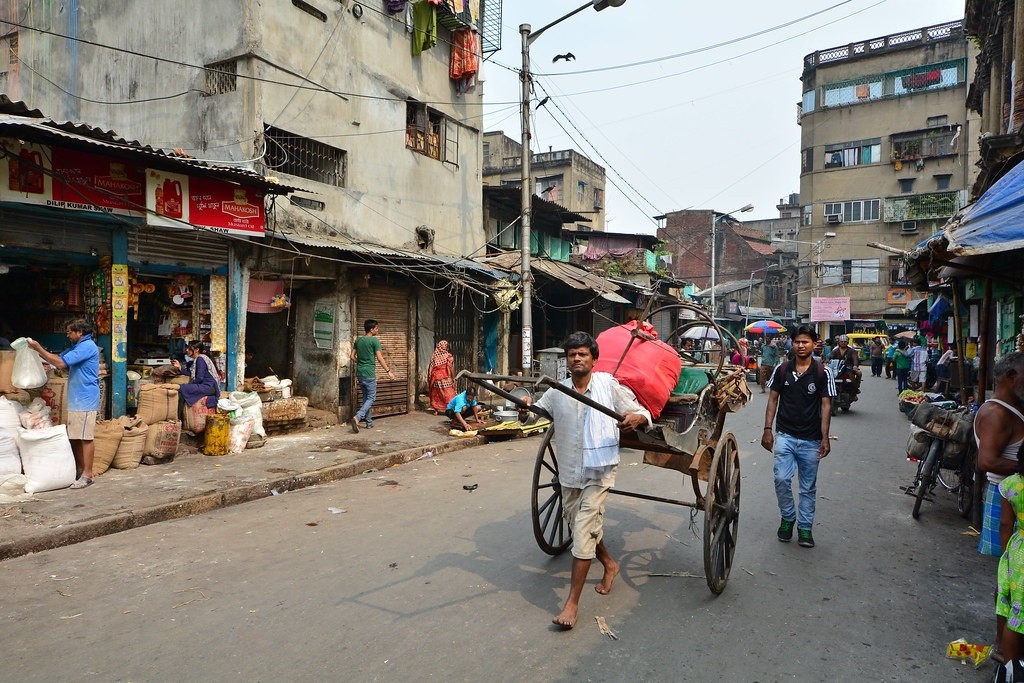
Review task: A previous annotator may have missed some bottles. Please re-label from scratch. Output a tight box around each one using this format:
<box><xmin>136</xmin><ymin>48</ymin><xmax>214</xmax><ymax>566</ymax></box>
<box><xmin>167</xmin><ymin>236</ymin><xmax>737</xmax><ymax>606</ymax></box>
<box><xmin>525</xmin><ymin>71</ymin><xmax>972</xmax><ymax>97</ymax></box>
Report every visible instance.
<box><xmin>9</xmin><ymin>158</ymin><xmax>19</xmax><ymax>190</ymax></box>
<box><xmin>155</xmin><ymin>184</ymin><xmax>164</xmax><ymax>215</ymax></box>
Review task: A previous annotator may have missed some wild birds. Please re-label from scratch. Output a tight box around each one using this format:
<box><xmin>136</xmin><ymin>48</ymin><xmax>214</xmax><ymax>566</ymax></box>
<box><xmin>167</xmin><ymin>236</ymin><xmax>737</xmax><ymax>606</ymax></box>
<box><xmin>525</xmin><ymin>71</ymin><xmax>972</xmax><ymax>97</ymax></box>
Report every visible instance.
<box><xmin>552</xmin><ymin>52</ymin><xmax>575</xmax><ymax>63</ymax></box>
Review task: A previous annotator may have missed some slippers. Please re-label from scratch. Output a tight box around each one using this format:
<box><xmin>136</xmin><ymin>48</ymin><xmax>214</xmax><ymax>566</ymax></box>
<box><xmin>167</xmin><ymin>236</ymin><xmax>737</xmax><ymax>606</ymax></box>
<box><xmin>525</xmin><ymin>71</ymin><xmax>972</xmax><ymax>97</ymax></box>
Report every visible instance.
<box><xmin>351</xmin><ymin>418</ymin><xmax>359</xmax><ymax>433</ymax></box>
<box><xmin>71</xmin><ymin>477</ymin><xmax>93</xmax><ymax>489</ymax></box>
<box><xmin>366</xmin><ymin>424</ymin><xmax>372</xmax><ymax>428</ymax></box>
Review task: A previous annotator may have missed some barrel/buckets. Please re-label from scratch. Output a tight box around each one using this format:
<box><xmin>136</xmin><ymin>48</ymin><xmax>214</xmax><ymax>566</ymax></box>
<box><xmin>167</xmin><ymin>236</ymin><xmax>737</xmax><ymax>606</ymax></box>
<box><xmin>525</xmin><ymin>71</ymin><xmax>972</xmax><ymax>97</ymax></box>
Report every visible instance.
<box><xmin>163</xmin><ymin>179</ymin><xmax>182</xmax><ymax>219</ymax></box>
<box><xmin>19</xmin><ymin>149</ymin><xmax>44</xmax><ymax>194</ymax></box>
<box><xmin>658</xmin><ymin>404</ymin><xmax>693</xmax><ymax>438</ymax></box>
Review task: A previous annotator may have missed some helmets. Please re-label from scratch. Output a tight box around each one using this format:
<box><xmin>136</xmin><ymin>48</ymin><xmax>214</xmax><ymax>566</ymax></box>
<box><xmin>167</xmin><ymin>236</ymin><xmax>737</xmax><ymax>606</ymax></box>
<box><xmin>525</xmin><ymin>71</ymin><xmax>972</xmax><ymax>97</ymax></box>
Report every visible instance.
<box><xmin>839</xmin><ymin>335</ymin><xmax>850</xmax><ymax>342</ymax></box>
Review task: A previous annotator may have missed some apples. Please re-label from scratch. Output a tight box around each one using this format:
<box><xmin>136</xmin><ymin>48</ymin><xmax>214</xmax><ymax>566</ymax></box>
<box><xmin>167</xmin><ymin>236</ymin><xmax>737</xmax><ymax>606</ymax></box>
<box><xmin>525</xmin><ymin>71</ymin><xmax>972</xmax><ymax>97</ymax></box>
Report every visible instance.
<box><xmin>904</xmin><ymin>394</ymin><xmax>928</xmax><ymax>405</ymax></box>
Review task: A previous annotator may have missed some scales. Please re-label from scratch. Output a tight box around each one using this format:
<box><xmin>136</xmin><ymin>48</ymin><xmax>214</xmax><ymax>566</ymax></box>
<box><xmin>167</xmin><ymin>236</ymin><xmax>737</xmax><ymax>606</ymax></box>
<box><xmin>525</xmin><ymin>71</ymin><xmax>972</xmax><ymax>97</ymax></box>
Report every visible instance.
<box><xmin>134</xmin><ymin>352</ymin><xmax>172</xmax><ymax>366</ymax></box>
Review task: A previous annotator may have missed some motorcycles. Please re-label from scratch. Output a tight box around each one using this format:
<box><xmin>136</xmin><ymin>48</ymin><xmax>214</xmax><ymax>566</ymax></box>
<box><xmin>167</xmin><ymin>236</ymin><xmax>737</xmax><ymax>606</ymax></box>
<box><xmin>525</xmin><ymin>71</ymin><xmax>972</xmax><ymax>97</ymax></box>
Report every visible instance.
<box><xmin>845</xmin><ymin>334</ymin><xmax>891</xmax><ymax>362</ymax></box>
<box><xmin>825</xmin><ymin>361</ymin><xmax>857</xmax><ymax>416</ymax></box>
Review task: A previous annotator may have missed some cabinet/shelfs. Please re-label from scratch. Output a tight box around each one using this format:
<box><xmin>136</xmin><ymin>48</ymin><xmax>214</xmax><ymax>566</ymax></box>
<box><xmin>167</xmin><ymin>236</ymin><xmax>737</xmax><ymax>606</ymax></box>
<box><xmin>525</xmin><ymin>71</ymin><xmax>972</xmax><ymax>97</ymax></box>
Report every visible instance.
<box><xmin>169</xmin><ymin>286</ymin><xmax>200</xmax><ymax>342</ymax></box>
<box><xmin>196</xmin><ymin>274</ymin><xmax>227</xmax><ymax>355</ymax></box>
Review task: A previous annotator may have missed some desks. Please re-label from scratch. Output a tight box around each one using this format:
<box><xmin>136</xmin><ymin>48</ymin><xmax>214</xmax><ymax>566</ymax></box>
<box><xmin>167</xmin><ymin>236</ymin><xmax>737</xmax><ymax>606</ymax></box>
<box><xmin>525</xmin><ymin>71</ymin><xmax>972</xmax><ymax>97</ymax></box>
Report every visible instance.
<box><xmin>127</xmin><ymin>364</ymin><xmax>165</xmax><ymax>384</ymax></box>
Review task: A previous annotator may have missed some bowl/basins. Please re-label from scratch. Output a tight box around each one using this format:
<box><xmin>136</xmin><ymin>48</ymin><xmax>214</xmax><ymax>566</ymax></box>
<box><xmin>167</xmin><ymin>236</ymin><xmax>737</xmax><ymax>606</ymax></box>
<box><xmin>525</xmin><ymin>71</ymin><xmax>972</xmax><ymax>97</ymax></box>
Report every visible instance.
<box><xmin>493</xmin><ymin>411</ymin><xmax>519</xmax><ymax>423</ymax></box>
<box><xmin>506</xmin><ymin>387</ymin><xmax>530</xmax><ymax>410</ymax></box>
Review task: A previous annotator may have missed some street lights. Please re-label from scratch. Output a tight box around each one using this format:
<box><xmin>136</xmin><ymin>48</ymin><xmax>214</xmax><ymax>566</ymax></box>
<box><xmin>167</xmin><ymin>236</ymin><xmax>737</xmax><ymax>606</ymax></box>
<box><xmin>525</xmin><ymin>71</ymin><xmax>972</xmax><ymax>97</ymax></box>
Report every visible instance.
<box><xmin>743</xmin><ymin>263</ymin><xmax>778</xmax><ymax>337</ymax></box>
<box><xmin>771</xmin><ymin>232</ymin><xmax>836</xmax><ymax>334</ymax></box>
<box><xmin>710</xmin><ymin>204</ymin><xmax>754</xmax><ymax>321</ymax></box>
<box><xmin>519</xmin><ymin>0</ymin><xmax>626</xmax><ymax>395</ymax></box>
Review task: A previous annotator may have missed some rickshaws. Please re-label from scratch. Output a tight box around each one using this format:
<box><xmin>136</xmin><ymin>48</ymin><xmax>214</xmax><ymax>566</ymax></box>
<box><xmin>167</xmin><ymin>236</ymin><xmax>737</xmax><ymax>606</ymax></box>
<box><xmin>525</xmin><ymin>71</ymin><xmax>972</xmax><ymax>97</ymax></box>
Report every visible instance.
<box><xmin>455</xmin><ymin>305</ymin><xmax>753</xmax><ymax>595</ymax></box>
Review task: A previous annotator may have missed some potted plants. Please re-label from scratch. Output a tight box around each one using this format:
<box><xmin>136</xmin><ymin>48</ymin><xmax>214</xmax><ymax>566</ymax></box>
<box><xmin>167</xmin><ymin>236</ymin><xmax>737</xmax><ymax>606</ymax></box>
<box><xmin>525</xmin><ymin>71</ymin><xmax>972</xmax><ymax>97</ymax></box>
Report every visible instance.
<box><xmin>901</xmin><ymin>140</ymin><xmax>922</xmax><ymax>160</ymax></box>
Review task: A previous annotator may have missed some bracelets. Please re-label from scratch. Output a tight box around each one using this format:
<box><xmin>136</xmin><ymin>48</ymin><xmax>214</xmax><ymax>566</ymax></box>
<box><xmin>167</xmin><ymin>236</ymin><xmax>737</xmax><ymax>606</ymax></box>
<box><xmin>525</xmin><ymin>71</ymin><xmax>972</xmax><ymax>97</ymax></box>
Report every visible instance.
<box><xmin>764</xmin><ymin>426</ymin><xmax>772</xmax><ymax>430</ymax></box>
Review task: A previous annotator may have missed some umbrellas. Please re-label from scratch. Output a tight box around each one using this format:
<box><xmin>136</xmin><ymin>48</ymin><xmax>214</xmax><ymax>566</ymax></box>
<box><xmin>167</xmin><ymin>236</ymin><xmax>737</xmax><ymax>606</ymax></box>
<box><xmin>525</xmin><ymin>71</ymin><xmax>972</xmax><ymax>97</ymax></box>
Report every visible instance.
<box><xmin>744</xmin><ymin>320</ymin><xmax>788</xmax><ymax>345</ymax></box>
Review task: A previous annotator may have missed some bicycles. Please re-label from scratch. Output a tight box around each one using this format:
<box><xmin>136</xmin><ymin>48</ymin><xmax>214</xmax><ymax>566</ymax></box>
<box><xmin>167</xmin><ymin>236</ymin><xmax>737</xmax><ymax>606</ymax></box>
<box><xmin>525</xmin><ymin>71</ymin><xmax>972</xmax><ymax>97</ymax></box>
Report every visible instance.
<box><xmin>898</xmin><ymin>404</ymin><xmax>976</xmax><ymax>518</ymax></box>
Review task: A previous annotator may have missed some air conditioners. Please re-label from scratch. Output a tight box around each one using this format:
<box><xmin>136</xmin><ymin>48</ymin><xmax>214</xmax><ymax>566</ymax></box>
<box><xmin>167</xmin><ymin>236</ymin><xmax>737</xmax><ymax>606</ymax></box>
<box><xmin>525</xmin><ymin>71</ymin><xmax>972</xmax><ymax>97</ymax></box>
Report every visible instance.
<box><xmin>902</xmin><ymin>221</ymin><xmax>917</xmax><ymax>231</ymax></box>
<box><xmin>827</xmin><ymin>214</ymin><xmax>843</xmax><ymax>223</ymax></box>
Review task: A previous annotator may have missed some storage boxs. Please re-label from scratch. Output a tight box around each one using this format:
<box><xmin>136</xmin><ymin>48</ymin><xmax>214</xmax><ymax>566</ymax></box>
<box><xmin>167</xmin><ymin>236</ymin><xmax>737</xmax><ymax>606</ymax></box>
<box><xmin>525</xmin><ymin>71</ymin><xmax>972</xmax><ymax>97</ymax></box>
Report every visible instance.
<box><xmin>127</xmin><ymin>380</ymin><xmax>154</xmax><ymax>408</ymax></box>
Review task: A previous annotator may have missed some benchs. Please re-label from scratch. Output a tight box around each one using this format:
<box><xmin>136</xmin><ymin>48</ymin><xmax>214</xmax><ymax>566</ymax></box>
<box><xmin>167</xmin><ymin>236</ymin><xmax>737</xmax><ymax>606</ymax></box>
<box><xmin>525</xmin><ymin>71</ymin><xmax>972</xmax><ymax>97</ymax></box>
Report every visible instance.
<box><xmin>661</xmin><ymin>368</ymin><xmax>710</xmax><ymax>432</ymax></box>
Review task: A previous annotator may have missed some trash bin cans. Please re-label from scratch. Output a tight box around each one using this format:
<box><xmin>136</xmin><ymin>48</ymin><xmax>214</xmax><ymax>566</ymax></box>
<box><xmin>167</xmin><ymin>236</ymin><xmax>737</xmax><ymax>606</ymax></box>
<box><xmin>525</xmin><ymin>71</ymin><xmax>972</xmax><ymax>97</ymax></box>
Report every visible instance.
<box><xmin>537</xmin><ymin>347</ymin><xmax>567</xmax><ymax>382</ymax></box>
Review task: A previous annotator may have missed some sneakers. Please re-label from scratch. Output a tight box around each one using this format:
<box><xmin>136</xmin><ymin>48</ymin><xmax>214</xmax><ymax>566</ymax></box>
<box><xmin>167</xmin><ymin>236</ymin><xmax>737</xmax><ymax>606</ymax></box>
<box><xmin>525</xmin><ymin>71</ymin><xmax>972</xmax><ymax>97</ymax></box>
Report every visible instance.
<box><xmin>797</xmin><ymin>526</ymin><xmax>815</xmax><ymax>548</ymax></box>
<box><xmin>778</xmin><ymin>519</ymin><xmax>795</xmax><ymax>542</ymax></box>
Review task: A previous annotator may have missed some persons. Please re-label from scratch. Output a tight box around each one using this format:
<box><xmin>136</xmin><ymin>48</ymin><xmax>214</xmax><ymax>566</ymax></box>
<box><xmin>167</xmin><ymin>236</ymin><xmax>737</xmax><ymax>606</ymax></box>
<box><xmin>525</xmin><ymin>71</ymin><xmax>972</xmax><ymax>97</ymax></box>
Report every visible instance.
<box><xmin>753</xmin><ymin>334</ymin><xmax>792</xmax><ymax>394</ymax></box>
<box><xmin>739</xmin><ymin>335</ymin><xmax>749</xmax><ymax>356</ymax></box>
<box><xmin>26</xmin><ymin>318</ymin><xmax>101</xmax><ymax>490</ymax></box>
<box><xmin>680</xmin><ymin>339</ymin><xmax>693</xmax><ymax>357</ymax></box>
<box><xmin>813</xmin><ymin>334</ymin><xmax>863</xmax><ymax>402</ymax></box>
<box><xmin>761</xmin><ymin>324</ymin><xmax>837</xmax><ymax>548</ymax></box>
<box><xmin>517</xmin><ymin>331</ymin><xmax>654</xmax><ymax>628</ymax></box>
<box><xmin>972</xmin><ymin>351</ymin><xmax>1024</xmax><ymax>683</ymax></box>
<box><xmin>445</xmin><ymin>387</ymin><xmax>487</xmax><ymax>431</ymax></box>
<box><xmin>936</xmin><ymin>345</ymin><xmax>958</xmax><ymax>394</ymax></box>
<box><xmin>870</xmin><ymin>337</ymin><xmax>929</xmax><ymax>396</ymax></box>
<box><xmin>428</xmin><ymin>340</ymin><xmax>456</xmax><ymax>415</ymax></box>
<box><xmin>350</xmin><ymin>319</ymin><xmax>394</xmax><ymax>433</ymax></box>
<box><xmin>170</xmin><ymin>340</ymin><xmax>221</xmax><ymax>419</ymax></box>
<box><xmin>694</xmin><ymin>336</ymin><xmax>728</xmax><ymax>363</ymax></box>
